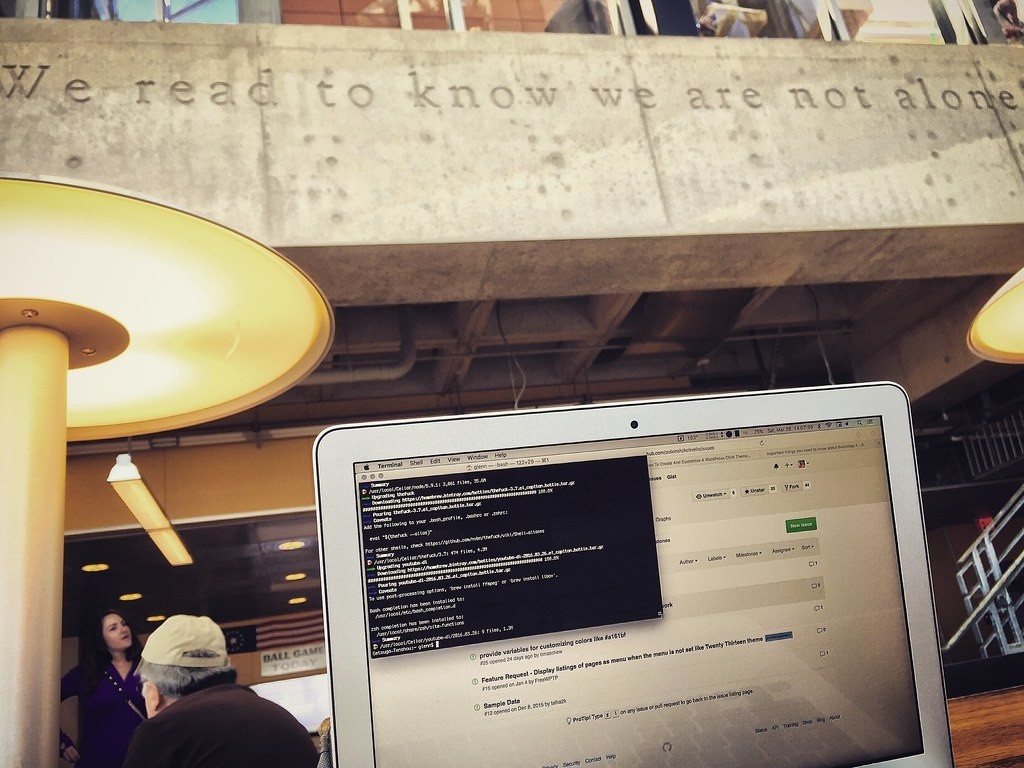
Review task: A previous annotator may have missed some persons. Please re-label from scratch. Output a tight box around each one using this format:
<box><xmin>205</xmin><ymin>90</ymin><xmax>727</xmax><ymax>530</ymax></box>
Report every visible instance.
<box><xmin>122</xmin><ymin>615</ymin><xmax>319</xmax><ymax>768</ymax></box>
<box><xmin>59</xmin><ymin>607</ymin><xmax>148</xmax><ymax>768</ymax></box>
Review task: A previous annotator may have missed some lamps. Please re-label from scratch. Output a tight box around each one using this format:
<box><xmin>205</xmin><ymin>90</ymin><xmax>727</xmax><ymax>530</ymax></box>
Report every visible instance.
<box><xmin>1</xmin><ymin>175</ymin><xmax>336</xmax><ymax>768</ymax></box>
<box><xmin>106</xmin><ymin>437</ymin><xmax>194</xmax><ymax>567</ymax></box>
<box><xmin>965</xmin><ymin>266</ymin><xmax>1024</xmax><ymax>365</ymax></box>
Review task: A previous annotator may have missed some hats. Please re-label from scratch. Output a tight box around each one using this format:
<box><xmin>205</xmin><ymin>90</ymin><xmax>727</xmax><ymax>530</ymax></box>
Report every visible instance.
<box><xmin>133</xmin><ymin>615</ymin><xmax>228</xmax><ymax>676</ymax></box>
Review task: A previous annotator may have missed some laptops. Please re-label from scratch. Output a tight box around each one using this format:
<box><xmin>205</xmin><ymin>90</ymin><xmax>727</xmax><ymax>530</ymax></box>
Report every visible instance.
<box><xmin>313</xmin><ymin>380</ymin><xmax>954</xmax><ymax>768</ymax></box>
